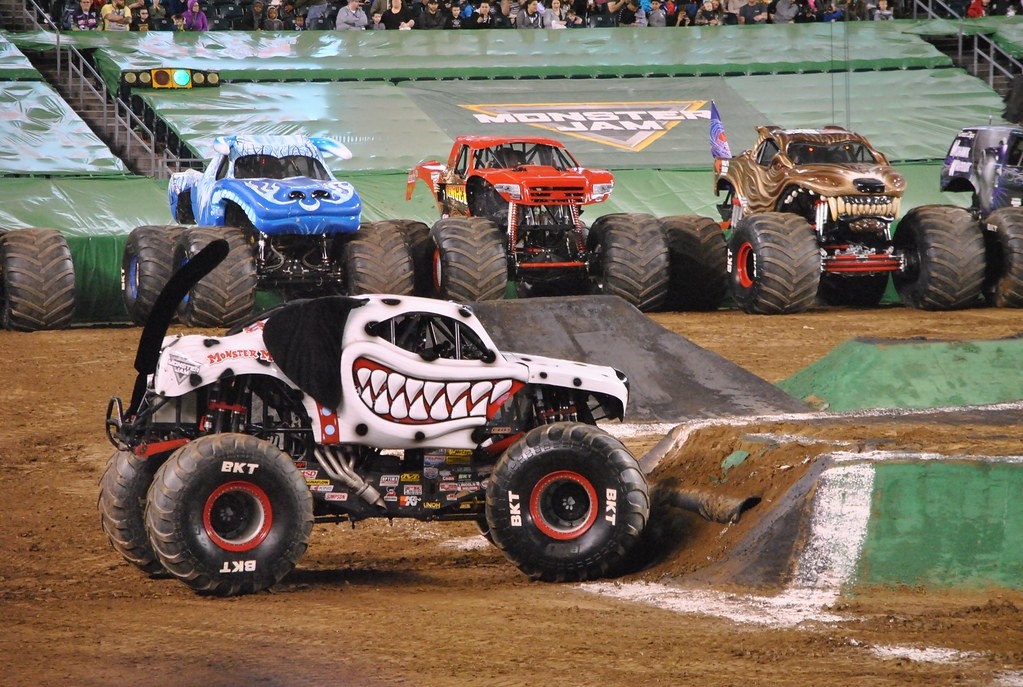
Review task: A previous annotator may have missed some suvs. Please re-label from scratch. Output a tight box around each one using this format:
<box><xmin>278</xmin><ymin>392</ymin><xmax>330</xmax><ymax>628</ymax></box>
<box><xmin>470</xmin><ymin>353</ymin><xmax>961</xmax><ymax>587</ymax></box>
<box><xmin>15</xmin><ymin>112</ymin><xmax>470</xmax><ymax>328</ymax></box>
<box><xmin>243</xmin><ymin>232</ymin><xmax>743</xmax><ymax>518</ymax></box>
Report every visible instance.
<box><xmin>387</xmin><ymin>137</ymin><xmax>673</xmax><ymax>314</ymax></box>
<box><xmin>96</xmin><ymin>238</ymin><xmax>651</xmax><ymax>598</ymax></box>
<box><xmin>120</xmin><ymin>140</ymin><xmax>407</xmax><ymax>327</ymax></box>
<box><xmin>936</xmin><ymin>124</ymin><xmax>1022</xmax><ymax>310</ymax></box>
<box><xmin>660</xmin><ymin>124</ymin><xmax>988</xmax><ymax>316</ymax></box>
<box><xmin>0</xmin><ymin>229</ymin><xmax>75</xmax><ymax>331</ymax></box>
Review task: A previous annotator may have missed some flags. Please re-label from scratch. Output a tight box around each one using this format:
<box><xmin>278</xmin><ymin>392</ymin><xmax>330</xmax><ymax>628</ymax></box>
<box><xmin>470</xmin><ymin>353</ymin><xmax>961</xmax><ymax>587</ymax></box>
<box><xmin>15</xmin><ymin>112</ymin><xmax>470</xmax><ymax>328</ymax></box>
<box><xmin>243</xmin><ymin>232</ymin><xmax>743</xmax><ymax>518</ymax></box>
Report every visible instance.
<box><xmin>709</xmin><ymin>104</ymin><xmax>732</xmax><ymax>159</ymax></box>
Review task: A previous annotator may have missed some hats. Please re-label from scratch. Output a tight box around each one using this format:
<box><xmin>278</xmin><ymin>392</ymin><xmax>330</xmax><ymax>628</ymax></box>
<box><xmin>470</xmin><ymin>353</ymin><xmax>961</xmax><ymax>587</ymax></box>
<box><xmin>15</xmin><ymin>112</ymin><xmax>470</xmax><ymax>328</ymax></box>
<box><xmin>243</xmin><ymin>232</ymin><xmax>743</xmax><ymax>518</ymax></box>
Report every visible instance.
<box><xmin>429</xmin><ymin>0</ymin><xmax>437</xmax><ymax>3</ymax></box>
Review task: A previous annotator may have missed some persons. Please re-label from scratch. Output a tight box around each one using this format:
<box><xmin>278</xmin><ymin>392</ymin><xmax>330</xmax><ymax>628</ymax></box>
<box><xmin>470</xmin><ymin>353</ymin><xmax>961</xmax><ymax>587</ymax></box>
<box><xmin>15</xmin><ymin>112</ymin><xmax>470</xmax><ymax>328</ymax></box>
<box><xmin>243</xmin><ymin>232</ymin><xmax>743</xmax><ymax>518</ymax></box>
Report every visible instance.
<box><xmin>968</xmin><ymin>0</ymin><xmax>990</xmax><ymax>18</ymax></box>
<box><xmin>35</xmin><ymin>0</ymin><xmax>893</xmax><ymax>31</ymax></box>
